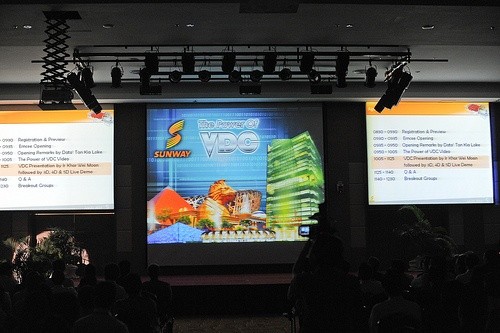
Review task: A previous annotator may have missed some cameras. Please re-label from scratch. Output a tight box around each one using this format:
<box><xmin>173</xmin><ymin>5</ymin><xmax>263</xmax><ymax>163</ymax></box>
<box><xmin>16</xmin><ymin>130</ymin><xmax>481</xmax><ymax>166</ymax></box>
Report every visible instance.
<box><xmin>298</xmin><ymin>224</ymin><xmax>321</xmax><ymax>239</ymax></box>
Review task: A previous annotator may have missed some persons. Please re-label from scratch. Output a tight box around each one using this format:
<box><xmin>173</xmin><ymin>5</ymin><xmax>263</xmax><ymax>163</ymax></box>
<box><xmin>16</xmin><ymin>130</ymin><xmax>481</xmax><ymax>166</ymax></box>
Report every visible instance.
<box><xmin>0</xmin><ymin>241</ymin><xmax>174</xmax><ymax>333</ymax></box>
<box><xmin>287</xmin><ymin>204</ymin><xmax>500</xmax><ymax>333</ymax></box>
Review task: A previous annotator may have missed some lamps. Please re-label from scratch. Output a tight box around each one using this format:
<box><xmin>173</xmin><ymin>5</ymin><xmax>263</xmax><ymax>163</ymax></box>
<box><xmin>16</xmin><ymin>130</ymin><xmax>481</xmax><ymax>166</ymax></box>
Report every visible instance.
<box><xmin>66</xmin><ymin>43</ymin><xmax>416</xmax><ymax>115</ymax></box>
